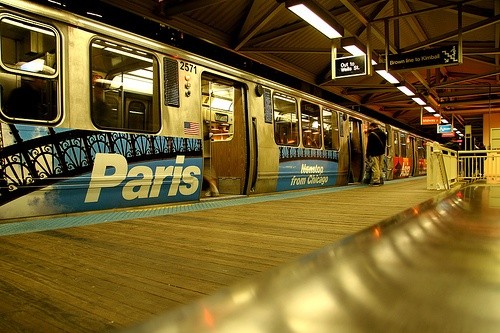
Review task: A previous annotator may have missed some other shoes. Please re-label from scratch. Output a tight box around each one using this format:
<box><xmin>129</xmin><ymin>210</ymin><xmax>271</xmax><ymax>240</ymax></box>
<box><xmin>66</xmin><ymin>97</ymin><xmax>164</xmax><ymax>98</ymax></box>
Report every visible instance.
<box><xmin>369</xmin><ymin>183</ymin><xmax>381</xmax><ymax>187</ymax></box>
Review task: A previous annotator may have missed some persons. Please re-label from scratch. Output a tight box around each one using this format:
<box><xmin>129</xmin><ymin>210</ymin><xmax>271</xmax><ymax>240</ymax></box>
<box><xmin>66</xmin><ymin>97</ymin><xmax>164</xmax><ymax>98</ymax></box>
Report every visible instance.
<box><xmin>474</xmin><ymin>141</ymin><xmax>487</xmax><ymax>177</ymax></box>
<box><xmin>365</xmin><ymin>122</ymin><xmax>387</xmax><ymax>187</ymax></box>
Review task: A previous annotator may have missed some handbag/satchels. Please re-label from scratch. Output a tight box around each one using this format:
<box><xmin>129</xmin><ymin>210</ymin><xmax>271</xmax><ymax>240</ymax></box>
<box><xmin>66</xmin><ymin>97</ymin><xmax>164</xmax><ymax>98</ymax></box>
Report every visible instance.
<box><xmin>365</xmin><ymin>158</ymin><xmax>375</xmax><ymax>173</ymax></box>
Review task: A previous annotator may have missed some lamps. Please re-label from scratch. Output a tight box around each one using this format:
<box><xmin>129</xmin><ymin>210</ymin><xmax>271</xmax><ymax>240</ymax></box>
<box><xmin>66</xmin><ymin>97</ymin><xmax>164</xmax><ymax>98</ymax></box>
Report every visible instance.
<box><xmin>285</xmin><ymin>1</ymin><xmax>481</xmax><ymax>151</ymax></box>
